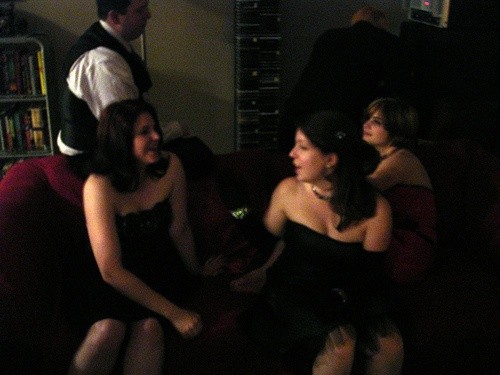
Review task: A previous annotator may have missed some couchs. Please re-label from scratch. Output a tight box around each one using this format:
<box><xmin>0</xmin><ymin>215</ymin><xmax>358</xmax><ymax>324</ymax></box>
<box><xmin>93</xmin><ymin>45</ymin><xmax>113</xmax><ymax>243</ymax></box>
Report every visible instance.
<box><xmin>1</xmin><ymin>134</ymin><xmax>500</xmax><ymax>375</ymax></box>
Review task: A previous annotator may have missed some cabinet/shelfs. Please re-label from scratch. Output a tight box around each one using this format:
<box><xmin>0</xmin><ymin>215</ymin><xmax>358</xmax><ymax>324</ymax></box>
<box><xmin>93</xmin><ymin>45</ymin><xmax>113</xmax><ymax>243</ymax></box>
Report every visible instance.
<box><xmin>0</xmin><ymin>33</ymin><xmax>58</xmax><ymax>179</ymax></box>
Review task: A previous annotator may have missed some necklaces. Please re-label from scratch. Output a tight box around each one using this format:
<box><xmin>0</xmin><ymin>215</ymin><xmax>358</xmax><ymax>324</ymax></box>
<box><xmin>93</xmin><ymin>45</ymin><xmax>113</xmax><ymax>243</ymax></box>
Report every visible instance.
<box><xmin>378</xmin><ymin>147</ymin><xmax>401</xmax><ymax>160</ymax></box>
<box><xmin>309</xmin><ymin>181</ymin><xmax>337</xmax><ymax>204</ymax></box>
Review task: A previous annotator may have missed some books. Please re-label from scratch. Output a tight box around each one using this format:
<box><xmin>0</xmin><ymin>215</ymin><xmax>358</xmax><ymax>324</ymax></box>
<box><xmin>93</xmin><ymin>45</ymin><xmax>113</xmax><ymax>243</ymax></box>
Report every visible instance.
<box><xmin>0</xmin><ymin>102</ymin><xmax>49</xmax><ymax>155</ymax></box>
<box><xmin>0</xmin><ymin>48</ymin><xmax>47</xmax><ymax>97</ymax></box>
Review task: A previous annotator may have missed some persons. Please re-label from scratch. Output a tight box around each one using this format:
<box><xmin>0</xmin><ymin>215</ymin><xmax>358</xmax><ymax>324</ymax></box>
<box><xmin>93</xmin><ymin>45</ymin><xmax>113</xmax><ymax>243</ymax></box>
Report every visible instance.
<box><xmin>356</xmin><ymin>96</ymin><xmax>443</xmax><ymax>290</ymax></box>
<box><xmin>65</xmin><ymin>97</ymin><xmax>230</xmax><ymax>374</ymax></box>
<box><xmin>58</xmin><ymin>0</ymin><xmax>190</xmax><ymax>178</ymax></box>
<box><xmin>217</xmin><ymin>113</ymin><xmax>406</xmax><ymax>375</ymax></box>
<box><xmin>282</xmin><ymin>5</ymin><xmax>413</xmax><ymax>171</ymax></box>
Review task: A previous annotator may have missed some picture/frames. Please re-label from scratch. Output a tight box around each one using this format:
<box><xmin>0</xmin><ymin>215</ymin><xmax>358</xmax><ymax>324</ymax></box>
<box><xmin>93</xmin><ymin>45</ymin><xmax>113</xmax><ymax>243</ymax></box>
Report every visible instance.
<box><xmin>130</xmin><ymin>32</ymin><xmax>145</xmax><ymax>65</ymax></box>
